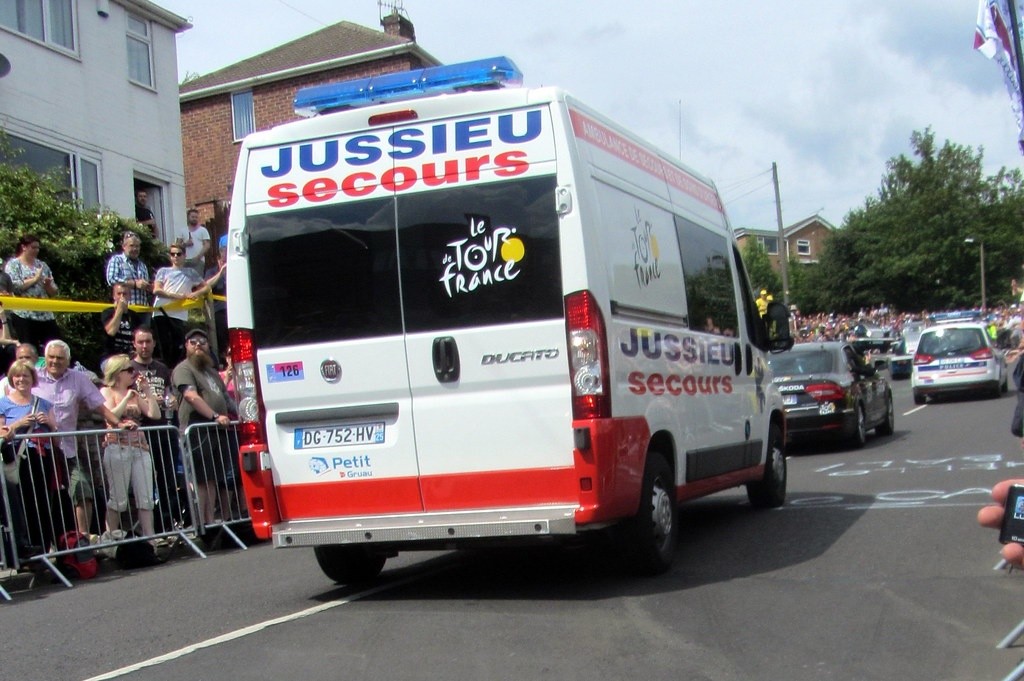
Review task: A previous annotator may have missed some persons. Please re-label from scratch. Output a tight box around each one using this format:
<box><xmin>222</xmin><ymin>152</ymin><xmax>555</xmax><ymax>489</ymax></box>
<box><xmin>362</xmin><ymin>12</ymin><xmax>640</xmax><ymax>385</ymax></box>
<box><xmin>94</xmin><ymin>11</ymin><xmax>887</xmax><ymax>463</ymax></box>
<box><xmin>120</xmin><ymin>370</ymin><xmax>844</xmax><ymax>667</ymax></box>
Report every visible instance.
<box><xmin>0</xmin><ymin>186</ymin><xmax>250</xmax><ymax>572</ymax></box>
<box><xmin>699</xmin><ymin>317</ymin><xmax>736</xmax><ymax>338</ymax></box>
<box><xmin>756</xmin><ymin>278</ymin><xmax>1024</xmax><ymax>573</ymax></box>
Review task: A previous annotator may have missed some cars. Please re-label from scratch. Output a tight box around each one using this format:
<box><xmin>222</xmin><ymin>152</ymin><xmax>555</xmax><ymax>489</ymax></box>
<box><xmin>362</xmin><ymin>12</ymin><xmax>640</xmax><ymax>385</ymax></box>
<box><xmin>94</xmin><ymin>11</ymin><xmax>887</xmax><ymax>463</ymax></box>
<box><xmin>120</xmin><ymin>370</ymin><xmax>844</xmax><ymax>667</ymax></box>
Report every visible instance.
<box><xmin>911</xmin><ymin>309</ymin><xmax>1010</xmax><ymax>405</ymax></box>
<box><xmin>864</xmin><ymin>320</ymin><xmax>922</xmax><ymax>380</ymax></box>
<box><xmin>765</xmin><ymin>341</ymin><xmax>894</xmax><ymax>456</ymax></box>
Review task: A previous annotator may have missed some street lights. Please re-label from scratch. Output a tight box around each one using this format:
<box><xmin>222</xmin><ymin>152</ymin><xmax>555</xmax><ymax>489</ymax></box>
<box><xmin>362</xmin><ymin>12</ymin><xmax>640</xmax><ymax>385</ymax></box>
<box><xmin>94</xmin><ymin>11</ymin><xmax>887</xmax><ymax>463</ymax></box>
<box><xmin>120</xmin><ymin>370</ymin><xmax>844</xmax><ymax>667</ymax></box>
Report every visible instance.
<box><xmin>964</xmin><ymin>237</ymin><xmax>987</xmax><ymax>316</ymax></box>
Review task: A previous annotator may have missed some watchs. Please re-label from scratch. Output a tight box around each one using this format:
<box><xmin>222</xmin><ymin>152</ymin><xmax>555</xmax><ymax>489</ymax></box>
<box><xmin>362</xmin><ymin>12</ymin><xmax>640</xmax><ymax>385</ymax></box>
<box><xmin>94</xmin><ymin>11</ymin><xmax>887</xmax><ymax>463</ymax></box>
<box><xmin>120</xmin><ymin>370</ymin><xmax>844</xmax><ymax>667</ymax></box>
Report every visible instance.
<box><xmin>211</xmin><ymin>412</ymin><xmax>219</xmax><ymax>422</ymax></box>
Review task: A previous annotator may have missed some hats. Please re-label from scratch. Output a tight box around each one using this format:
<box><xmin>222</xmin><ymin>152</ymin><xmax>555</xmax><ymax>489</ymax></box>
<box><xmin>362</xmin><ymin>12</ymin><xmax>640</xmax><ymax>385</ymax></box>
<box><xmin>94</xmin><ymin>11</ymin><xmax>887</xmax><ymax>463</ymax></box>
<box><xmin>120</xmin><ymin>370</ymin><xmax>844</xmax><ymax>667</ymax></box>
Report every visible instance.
<box><xmin>184</xmin><ymin>328</ymin><xmax>210</xmax><ymax>341</ymax></box>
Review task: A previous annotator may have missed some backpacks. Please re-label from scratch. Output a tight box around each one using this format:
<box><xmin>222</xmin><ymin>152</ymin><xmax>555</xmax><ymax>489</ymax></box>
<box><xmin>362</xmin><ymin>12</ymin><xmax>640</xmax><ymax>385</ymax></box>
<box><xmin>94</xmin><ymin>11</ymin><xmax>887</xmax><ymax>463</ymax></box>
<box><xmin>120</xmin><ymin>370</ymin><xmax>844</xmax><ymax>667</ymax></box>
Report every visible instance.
<box><xmin>51</xmin><ymin>527</ymin><xmax>98</xmax><ymax>586</ymax></box>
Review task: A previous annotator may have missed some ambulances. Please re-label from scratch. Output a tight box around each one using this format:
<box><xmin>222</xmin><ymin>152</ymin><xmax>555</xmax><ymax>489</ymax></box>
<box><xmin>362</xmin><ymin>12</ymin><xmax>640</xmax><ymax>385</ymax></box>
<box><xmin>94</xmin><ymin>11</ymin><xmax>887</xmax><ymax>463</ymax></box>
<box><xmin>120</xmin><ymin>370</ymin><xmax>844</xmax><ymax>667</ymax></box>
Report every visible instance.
<box><xmin>226</xmin><ymin>55</ymin><xmax>797</xmax><ymax>592</ymax></box>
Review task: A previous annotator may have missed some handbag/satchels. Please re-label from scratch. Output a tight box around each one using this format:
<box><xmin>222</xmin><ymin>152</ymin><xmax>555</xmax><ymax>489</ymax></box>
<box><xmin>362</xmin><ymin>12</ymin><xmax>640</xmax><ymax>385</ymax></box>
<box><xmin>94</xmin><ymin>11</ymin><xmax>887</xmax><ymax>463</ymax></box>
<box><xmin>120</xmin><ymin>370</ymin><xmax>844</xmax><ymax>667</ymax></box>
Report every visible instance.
<box><xmin>2</xmin><ymin>458</ymin><xmax>20</xmax><ymax>486</ymax></box>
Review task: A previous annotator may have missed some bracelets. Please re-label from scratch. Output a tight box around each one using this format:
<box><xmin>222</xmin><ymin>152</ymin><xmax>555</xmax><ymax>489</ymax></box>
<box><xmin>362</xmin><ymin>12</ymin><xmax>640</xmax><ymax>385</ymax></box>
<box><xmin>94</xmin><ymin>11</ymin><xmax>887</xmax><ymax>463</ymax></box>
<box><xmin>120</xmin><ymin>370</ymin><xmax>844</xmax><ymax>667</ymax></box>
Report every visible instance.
<box><xmin>2</xmin><ymin>320</ymin><xmax>7</xmax><ymax>324</ymax></box>
<box><xmin>116</xmin><ymin>421</ymin><xmax>122</xmax><ymax>426</ymax></box>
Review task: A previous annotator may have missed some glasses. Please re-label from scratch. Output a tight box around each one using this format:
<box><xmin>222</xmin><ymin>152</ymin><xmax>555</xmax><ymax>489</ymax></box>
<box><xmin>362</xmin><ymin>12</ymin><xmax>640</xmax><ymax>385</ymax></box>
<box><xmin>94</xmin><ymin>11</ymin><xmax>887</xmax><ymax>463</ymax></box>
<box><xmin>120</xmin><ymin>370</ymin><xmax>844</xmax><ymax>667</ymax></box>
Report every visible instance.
<box><xmin>189</xmin><ymin>337</ymin><xmax>206</xmax><ymax>346</ymax></box>
<box><xmin>170</xmin><ymin>252</ymin><xmax>182</xmax><ymax>257</ymax></box>
<box><xmin>120</xmin><ymin>367</ymin><xmax>135</xmax><ymax>375</ymax></box>
<box><xmin>125</xmin><ymin>233</ymin><xmax>140</xmax><ymax>240</ymax></box>
